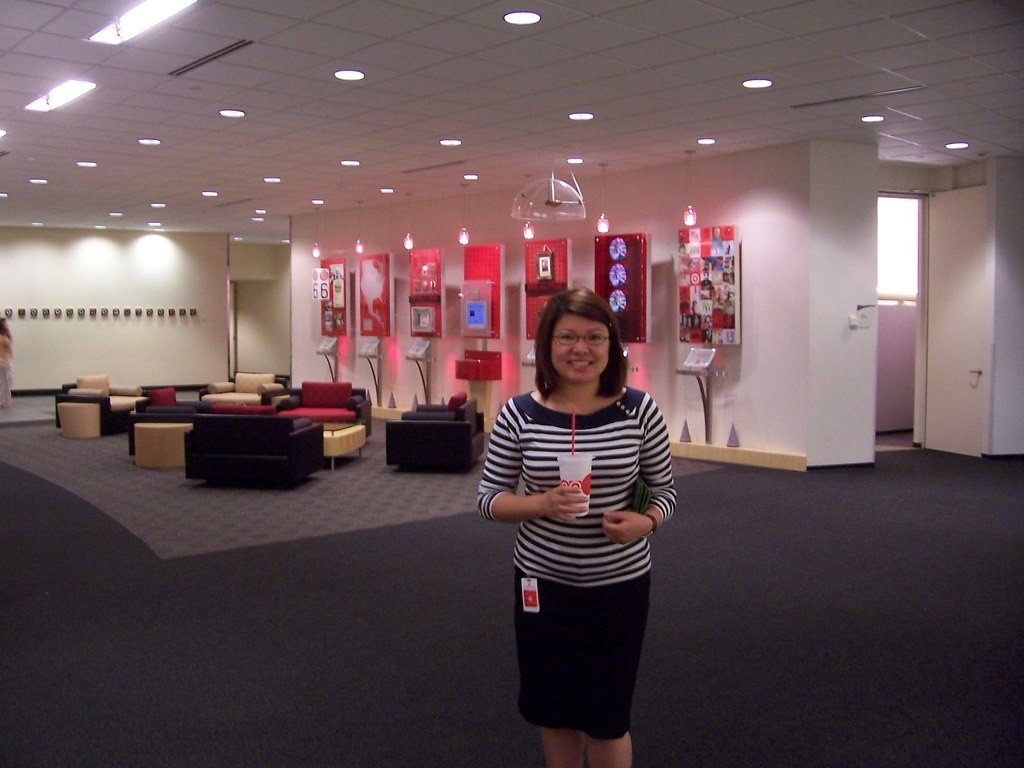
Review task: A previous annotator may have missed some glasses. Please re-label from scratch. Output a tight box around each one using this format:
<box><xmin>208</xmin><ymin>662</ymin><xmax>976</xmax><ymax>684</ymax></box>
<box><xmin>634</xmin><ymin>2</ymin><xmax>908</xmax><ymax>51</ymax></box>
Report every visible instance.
<box><xmin>552</xmin><ymin>332</ymin><xmax>610</xmax><ymax>345</ymax></box>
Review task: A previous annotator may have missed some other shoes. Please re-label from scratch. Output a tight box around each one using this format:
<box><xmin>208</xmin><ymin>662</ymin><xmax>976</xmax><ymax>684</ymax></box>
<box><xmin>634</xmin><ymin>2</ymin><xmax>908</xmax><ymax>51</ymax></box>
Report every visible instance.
<box><xmin>2</xmin><ymin>403</ymin><xmax>7</xmax><ymax>407</ymax></box>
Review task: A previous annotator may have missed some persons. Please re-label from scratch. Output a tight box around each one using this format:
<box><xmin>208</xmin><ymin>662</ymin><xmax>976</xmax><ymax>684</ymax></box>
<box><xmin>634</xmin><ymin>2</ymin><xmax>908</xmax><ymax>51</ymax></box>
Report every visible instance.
<box><xmin>0</xmin><ymin>317</ymin><xmax>13</xmax><ymax>408</ymax></box>
<box><xmin>679</xmin><ymin>227</ymin><xmax>734</xmax><ymax>344</ymax></box>
<box><xmin>476</xmin><ymin>288</ymin><xmax>677</xmax><ymax>768</ymax></box>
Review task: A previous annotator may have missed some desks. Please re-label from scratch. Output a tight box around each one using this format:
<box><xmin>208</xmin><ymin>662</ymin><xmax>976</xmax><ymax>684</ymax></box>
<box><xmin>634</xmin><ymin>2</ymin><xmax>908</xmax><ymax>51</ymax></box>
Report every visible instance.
<box><xmin>323</xmin><ymin>423</ymin><xmax>368</xmax><ymax>472</ymax></box>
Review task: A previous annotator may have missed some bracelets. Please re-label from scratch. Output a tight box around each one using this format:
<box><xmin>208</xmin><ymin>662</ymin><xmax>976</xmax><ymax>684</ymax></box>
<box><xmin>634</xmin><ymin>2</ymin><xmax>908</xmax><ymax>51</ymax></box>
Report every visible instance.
<box><xmin>642</xmin><ymin>513</ymin><xmax>657</xmax><ymax>537</ymax></box>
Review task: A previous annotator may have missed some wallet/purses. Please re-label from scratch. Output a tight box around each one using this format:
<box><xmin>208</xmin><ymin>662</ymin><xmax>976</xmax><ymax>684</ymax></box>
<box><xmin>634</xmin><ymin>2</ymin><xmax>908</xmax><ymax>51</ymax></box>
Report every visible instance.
<box><xmin>632</xmin><ymin>477</ymin><xmax>657</xmax><ymax>514</ymax></box>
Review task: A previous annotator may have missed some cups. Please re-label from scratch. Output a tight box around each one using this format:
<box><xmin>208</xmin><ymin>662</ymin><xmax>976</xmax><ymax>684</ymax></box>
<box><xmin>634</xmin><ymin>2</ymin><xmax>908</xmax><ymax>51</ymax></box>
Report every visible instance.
<box><xmin>558</xmin><ymin>453</ymin><xmax>592</xmax><ymax>517</ymax></box>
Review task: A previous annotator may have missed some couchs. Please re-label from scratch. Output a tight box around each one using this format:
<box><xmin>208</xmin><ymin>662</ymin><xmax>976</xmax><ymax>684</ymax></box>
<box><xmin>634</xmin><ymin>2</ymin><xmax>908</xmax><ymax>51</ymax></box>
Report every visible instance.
<box><xmin>386</xmin><ymin>391</ymin><xmax>485</xmax><ymax>470</ymax></box>
<box><xmin>54</xmin><ymin>374</ymin><xmax>373</xmax><ymax>491</ymax></box>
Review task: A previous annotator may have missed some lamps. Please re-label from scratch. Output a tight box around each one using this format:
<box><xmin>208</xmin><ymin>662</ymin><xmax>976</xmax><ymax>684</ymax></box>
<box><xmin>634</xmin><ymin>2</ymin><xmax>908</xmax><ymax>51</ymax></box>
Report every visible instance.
<box><xmin>312</xmin><ymin>205</ymin><xmax>321</xmax><ymax>257</ymax></box>
<box><xmin>523</xmin><ymin>221</ymin><xmax>534</xmax><ymax>239</ymax></box>
<box><xmin>403</xmin><ymin>193</ymin><xmax>414</xmax><ymax>249</ymax></box>
<box><xmin>597</xmin><ymin>162</ymin><xmax>609</xmax><ymax>233</ymax></box>
<box><xmin>355</xmin><ymin>199</ymin><xmax>364</xmax><ymax>252</ymax></box>
<box><xmin>683</xmin><ymin>149</ymin><xmax>697</xmax><ymax>226</ymax></box>
<box><xmin>458</xmin><ymin>184</ymin><xmax>470</xmax><ymax>245</ymax></box>
